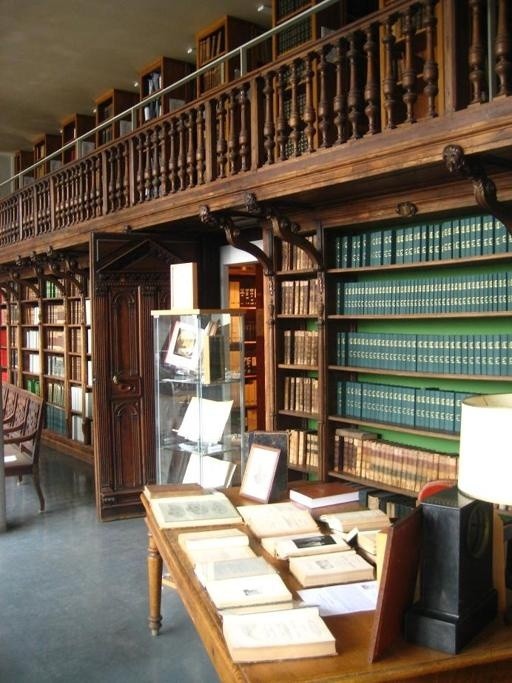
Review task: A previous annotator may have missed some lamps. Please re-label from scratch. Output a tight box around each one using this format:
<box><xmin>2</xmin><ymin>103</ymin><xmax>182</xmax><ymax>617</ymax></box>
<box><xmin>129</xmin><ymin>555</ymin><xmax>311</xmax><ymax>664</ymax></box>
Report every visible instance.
<box><xmin>456</xmin><ymin>394</ymin><xmax>512</xmax><ymax>511</ymax></box>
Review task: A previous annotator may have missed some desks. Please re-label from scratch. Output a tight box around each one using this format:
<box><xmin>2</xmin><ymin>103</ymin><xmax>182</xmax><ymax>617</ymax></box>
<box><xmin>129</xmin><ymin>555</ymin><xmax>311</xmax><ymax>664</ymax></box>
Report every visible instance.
<box><xmin>140</xmin><ymin>480</ymin><xmax>512</xmax><ymax>683</ymax></box>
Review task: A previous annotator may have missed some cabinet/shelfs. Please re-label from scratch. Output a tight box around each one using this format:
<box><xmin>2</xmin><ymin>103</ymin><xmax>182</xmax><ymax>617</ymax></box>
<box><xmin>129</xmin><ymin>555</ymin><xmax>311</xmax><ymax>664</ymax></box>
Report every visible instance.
<box><xmin>271</xmin><ymin>0</ymin><xmax>319</xmax><ymax>162</ymax></box>
<box><xmin>138</xmin><ymin>56</ymin><xmax>195</xmax><ymax>127</ymax></box>
<box><xmin>262</xmin><ymin>212</ymin><xmax>322</xmax><ymax>483</ymax></box>
<box><xmin>0</xmin><ymin>263</ymin><xmax>95</xmax><ymax>466</ymax></box>
<box><xmin>227</xmin><ymin>263</ymin><xmax>258</xmax><ymax>433</ymax></box>
<box><xmin>95</xmin><ymin>87</ymin><xmax>139</xmax><ymax>150</ymax></box>
<box><xmin>378</xmin><ymin>0</ymin><xmax>444</xmax><ymax>132</ymax></box>
<box><xmin>322</xmin><ymin>174</ymin><xmax>511</xmax><ymax>520</ymax></box>
<box><xmin>195</xmin><ymin>14</ymin><xmax>269</xmax><ymax>98</ymax></box>
<box><xmin>151</xmin><ymin>308</ymin><xmax>248</xmax><ymax>488</ymax></box>
<box><xmin>61</xmin><ymin>113</ymin><xmax>95</xmax><ymax>165</ymax></box>
<box><xmin>10</xmin><ymin>133</ymin><xmax>63</xmax><ymax>193</ymax></box>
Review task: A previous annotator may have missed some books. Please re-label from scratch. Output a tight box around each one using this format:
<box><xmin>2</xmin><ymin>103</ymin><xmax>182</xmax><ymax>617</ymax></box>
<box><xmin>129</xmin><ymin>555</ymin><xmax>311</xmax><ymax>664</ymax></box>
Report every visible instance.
<box><xmin>0</xmin><ymin>272</ymin><xmax>94</xmax><ymax>446</ymax></box>
<box><xmin>37</xmin><ymin>0</ymin><xmax>437</xmax><ymax>177</ymax></box>
<box><xmin>150</xmin><ymin>491</ymin><xmax>394</xmax><ymax>663</ymax></box>
<box><xmin>166</xmin><ymin>215</ymin><xmax>511</xmax><ymax>494</ymax></box>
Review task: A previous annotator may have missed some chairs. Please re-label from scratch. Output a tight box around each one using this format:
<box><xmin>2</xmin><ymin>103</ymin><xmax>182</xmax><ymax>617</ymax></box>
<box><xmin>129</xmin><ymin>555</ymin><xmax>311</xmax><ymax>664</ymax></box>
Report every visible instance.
<box><xmin>2</xmin><ymin>383</ymin><xmax>46</xmax><ymax>512</ymax></box>
